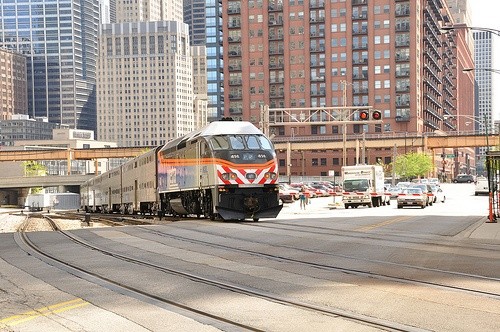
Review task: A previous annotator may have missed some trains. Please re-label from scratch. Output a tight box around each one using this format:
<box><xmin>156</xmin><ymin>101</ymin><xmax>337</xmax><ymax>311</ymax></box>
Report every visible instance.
<box><xmin>80</xmin><ymin>116</ymin><xmax>284</xmax><ymax>222</ymax></box>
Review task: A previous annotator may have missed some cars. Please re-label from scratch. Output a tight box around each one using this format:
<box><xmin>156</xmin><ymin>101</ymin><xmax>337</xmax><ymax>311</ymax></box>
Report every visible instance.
<box><xmin>383</xmin><ymin>177</ymin><xmax>446</xmax><ymax>206</ymax></box>
<box><xmin>473</xmin><ymin>174</ymin><xmax>500</xmax><ymax>196</ymax></box>
<box><xmin>397</xmin><ymin>188</ymin><xmax>427</xmax><ymax>209</ymax></box>
<box><xmin>453</xmin><ymin>175</ymin><xmax>474</xmax><ymax>183</ymax></box>
<box><xmin>278</xmin><ymin>180</ymin><xmax>343</xmax><ymax>206</ymax></box>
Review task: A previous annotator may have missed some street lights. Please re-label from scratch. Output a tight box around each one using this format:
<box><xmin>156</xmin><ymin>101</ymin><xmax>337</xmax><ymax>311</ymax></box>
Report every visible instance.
<box><xmin>443</xmin><ymin>114</ymin><xmax>488</xmax><ymax>149</ymax></box>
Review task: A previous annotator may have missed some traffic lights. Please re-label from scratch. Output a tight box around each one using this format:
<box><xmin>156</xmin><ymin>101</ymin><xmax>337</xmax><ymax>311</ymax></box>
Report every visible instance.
<box><xmin>373</xmin><ymin>111</ymin><xmax>381</xmax><ymax>120</ymax></box>
<box><xmin>360</xmin><ymin>111</ymin><xmax>369</xmax><ymax>120</ymax></box>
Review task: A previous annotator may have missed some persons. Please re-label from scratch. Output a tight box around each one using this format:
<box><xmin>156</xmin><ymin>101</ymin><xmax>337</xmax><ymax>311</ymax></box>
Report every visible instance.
<box><xmin>299</xmin><ymin>184</ymin><xmax>311</xmax><ymax>210</ymax></box>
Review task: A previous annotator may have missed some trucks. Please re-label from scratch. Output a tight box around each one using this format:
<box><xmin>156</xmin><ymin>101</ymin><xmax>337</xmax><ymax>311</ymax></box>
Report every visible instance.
<box><xmin>341</xmin><ymin>165</ymin><xmax>384</xmax><ymax>209</ymax></box>
